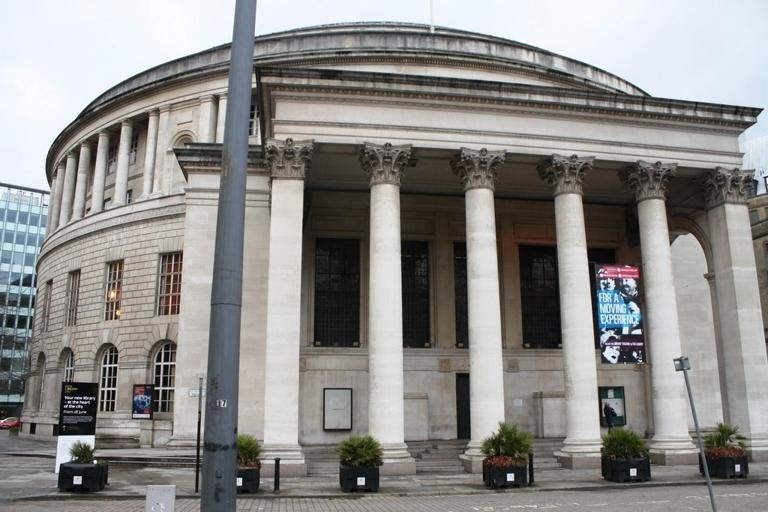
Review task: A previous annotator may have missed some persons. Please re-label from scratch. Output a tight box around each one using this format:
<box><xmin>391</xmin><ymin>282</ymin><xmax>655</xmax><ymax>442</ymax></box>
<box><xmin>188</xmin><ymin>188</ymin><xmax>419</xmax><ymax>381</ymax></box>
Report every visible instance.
<box><xmin>598</xmin><ymin>276</ymin><xmax>643</xmax><ymax>364</ymax></box>
<box><xmin>603</xmin><ymin>402</ymin><xmax>616</xmax><ymax>435</ymax></box>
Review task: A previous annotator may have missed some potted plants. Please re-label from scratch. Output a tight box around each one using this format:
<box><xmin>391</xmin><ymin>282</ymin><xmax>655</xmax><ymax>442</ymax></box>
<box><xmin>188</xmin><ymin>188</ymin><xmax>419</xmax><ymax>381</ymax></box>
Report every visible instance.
<box><xmin>335</xmin><ymin>433</ymin><xmax>385</xmax><ymax>493</ymax></box>
<box><xmin>236</xmin><ymin>431</ymin><xmax>265</xmax><ymax>494</ymax></box>
<box><xmin>699</xmin><ymin>421</ymin><xmax>750</xmax><ymax>480</ymax></box>
<box><xmin>599</xmin><ymin>425</ymin><xmax>654</xmax><ymax>481</ymax></box>
<box><xmin>57</xmin><ymin>441</ymin><xmax>109</xmax><ymax>493</ymax></box>
<box><xmin>482</xmin><ymin>420</ymin><xmax>533</xmax><ymax>488</ymax></box>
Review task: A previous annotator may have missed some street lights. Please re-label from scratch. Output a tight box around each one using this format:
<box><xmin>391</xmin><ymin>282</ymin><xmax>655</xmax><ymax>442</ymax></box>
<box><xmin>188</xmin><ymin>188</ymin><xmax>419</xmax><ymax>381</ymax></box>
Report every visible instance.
<box><xmin>672</xmin><ymin>354</ymin><xmax>717</xmax><ymax>511</ymax></box>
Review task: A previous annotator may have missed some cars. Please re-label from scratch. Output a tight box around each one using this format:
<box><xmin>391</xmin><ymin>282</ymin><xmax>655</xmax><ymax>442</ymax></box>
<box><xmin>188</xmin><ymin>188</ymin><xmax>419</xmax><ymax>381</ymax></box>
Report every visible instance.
<box><xmin>0</xmin><ymin>416</ymin><xmax>20</xmax><ymax>429</ymax></box>
